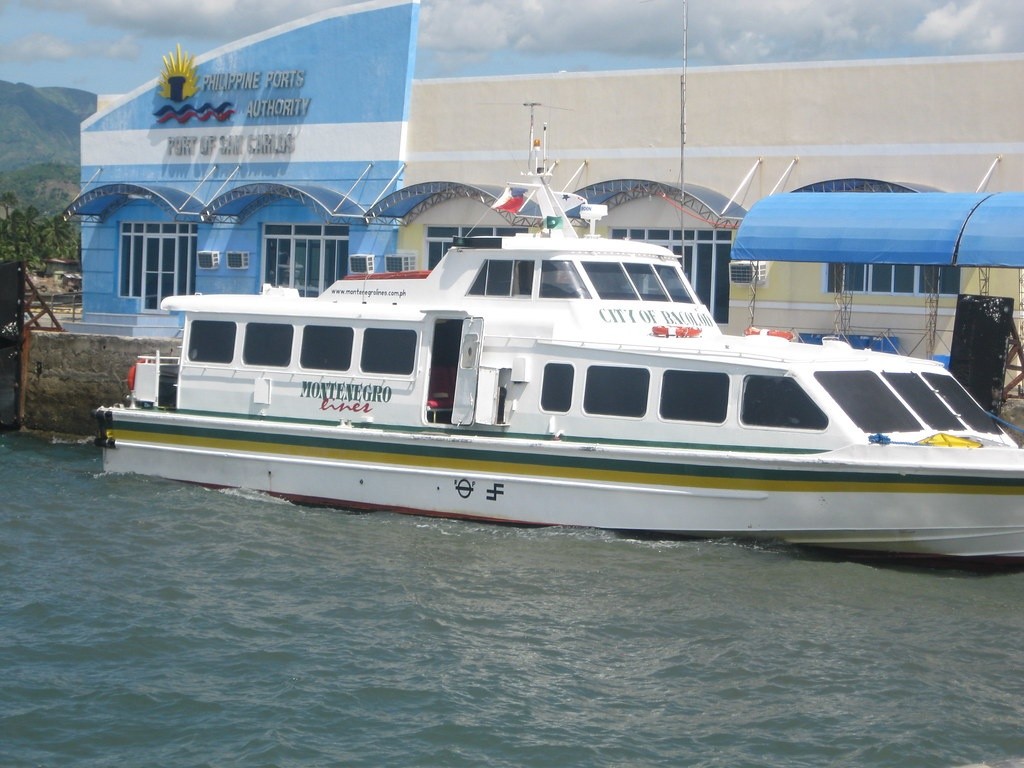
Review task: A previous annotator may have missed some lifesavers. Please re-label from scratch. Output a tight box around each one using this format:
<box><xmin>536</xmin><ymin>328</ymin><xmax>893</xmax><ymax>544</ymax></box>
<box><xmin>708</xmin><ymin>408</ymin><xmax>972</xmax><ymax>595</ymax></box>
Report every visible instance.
<box><xmin>126</xmin><ymin>356</ymin><xmax>159</xmax><ymax>391</ymax></box>
<box><xmin>649</xmin><ymin>322</ymin><xmax>705</xmax><ymax>339</ymax></box>
<box><xmin>743</xmin><ymin>325</ymin><xmax>796</xmax><ymax>342</ymax></box>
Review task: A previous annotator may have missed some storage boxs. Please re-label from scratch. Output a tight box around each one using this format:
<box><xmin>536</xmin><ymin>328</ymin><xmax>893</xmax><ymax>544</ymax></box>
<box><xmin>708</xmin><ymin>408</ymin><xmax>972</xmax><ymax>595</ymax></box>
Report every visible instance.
<box><xmin>799</xmin><ymin>333</ymin><xmax>899</xmax><ymax>354</ymax></box>
<box><xmin>931</xmin><ymin>354</ymin><xmax>949</xmax><ymax>371</ymax></box>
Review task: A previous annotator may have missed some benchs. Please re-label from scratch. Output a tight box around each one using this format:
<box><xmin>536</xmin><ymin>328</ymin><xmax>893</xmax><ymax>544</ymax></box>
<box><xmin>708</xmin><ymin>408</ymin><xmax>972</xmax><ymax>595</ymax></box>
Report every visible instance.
<box><xmin>427</xmin><ymin>367</ymin><xmax>458</xmax><ymax>422</ymax></box>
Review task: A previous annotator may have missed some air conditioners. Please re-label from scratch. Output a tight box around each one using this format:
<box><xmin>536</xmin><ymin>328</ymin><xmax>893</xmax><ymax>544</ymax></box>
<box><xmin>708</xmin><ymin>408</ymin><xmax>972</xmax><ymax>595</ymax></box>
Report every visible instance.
<box><xmin>384</xmin><ymin>253</ymin><xmax>418</xmax><ymax>272</ymax></box>
<box><xmin>197</xmin><ymin>250</ymin><xmax>220</xmax><ymax>269</ymax></box>
<box><xmin>227</xmin><ymin>251</ymin><xmax>249</xmax><ymax>270</ymax></box>
<box><xmin>350</xmin><ymin>254</ymin><xmax>375</xmax><ymax>274</ymax></box>
<box><xmin>728</xmin><ymin>261</ymin><xmax>767</xmax><ymax>285</ymax></box>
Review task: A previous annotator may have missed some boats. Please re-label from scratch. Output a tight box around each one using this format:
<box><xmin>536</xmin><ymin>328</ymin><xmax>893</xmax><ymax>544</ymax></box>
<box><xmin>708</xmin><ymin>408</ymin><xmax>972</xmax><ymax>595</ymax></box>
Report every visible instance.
<box><xmin>91</xmin><ymin>97</ymin><xmax>1024</xmax><ymax>561</ymax></box>
